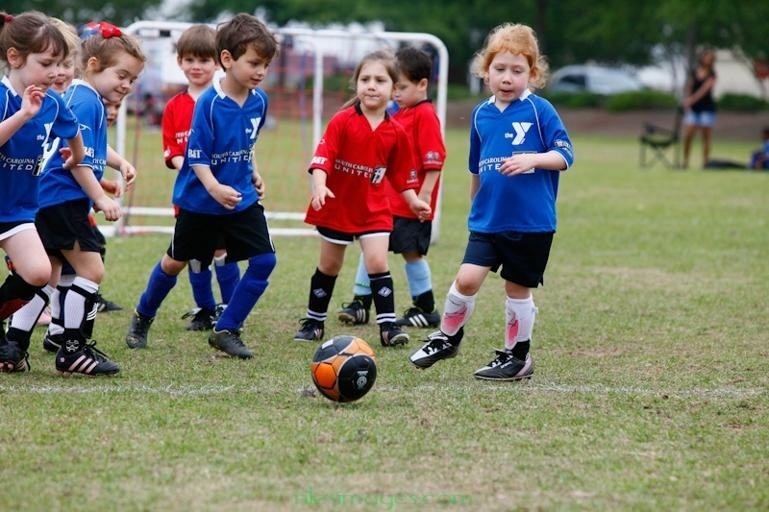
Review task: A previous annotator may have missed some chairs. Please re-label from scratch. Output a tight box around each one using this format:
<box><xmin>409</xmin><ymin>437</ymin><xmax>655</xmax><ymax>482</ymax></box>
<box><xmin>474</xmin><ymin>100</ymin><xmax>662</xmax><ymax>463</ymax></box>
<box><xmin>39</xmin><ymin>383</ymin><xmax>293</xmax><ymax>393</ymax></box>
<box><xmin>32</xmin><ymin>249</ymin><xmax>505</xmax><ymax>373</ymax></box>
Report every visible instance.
<box><xmin>636</xmin><ymin>106</ymin><xmax>684</xmax><ymax>171</ymax></box>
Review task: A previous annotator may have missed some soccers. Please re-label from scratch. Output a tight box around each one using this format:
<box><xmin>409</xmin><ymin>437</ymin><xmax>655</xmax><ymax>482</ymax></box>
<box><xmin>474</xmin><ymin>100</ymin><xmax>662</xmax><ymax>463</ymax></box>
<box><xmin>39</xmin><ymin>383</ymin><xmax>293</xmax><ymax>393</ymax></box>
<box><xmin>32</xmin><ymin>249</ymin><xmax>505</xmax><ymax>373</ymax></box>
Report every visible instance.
<box><xmin>311</xmin><ymin>335</ymin><xmax>377</xmax><ymax>403</ymax></box>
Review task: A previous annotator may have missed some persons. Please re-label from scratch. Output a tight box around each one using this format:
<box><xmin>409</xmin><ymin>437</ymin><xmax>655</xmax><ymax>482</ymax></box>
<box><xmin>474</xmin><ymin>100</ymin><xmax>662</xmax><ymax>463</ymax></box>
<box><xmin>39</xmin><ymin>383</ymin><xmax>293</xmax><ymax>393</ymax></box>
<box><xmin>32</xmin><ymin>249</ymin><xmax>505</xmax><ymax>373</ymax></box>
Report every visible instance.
<box><xmin>683</xmin><ymin>43</ymin><xmax>719</xmax><ymax>166</ymax></box>
<box><xmin>406</xmin><ymin>18</ymin><xmax>577</xmax><ymax>381</ymax></box>
<box><xmin>125</xmin><ymin>13</ymin><xmax>279</xmax><ymax>360</ymax></box>
<box><xmin>333</xmin><ymin>50</ymin><xmax>444</xmax><ymax>334</ymax></box>
<box><xmin>1</xmin><ymin>11</ymin><xmax>147</xmax><ymax>376</ymax></box>
<box><xmin>296</xmin><ymin>48</ymin><xmax>415</xmax><ymax>349</ymax></box>
<box><xmin>160</xmin><ymin>25</ymin><xmax>245</xmax><ymax>331</ymax></box>
<box><xmin>751</xmin><ymin>128</ymin><xmax>768</xmax><ymax>172</ymax></box>
<box><xmin>136</xmin><ymin>91</ymin><xmax>164</xmax><ymax>125</ymax></box>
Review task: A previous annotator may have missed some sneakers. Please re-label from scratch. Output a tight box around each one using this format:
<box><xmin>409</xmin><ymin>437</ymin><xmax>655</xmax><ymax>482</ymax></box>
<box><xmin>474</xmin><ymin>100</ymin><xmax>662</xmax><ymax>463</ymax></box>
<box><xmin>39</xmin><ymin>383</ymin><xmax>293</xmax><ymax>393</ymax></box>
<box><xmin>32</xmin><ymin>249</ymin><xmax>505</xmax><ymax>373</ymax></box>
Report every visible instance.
<box><xmin>181</xmin><ymin>307</ymin><xmax>216</xmax><ymax>331</ymax></box>
<box><xmin>295</xmin><ymin>318</ymin><xmax>324</xmax><ymax>342</ymax></box>
<box><xmin>97</xmin><ymin>295</ymin><xmax>123</xmax><ymax>312</ymax></box>
<box><xmin>211</xmin><ymin>303</ymin><xmax>228</xmax><ymax>326</ymax></box>
<box><xmin>0</xmin><ymin>340</ymin><xmax>31</xmax><ymax>372</ymax></box>
<box><xmin>43</xmin><ymin>328</ymin><xmax>63</xmax><ymax>353</ymax></box>
<box><xmin>208</xmin><ymin>326</ymin><xmax>252</xmax><ymax>360</ymax></box>
<box><xmin>126</xmin><ymin>308</ymin><xmax>155</xmax><ymax>348</ymax></box>
<box><xmin>55</xmin><ymin>340</ymin><xmax>119</xmax><ymax>376</ymax></box>
<box><xmin>337</xmin><ymin>300</ymin><xmax>369</xmax><ymax>325</ymax></box>
<box><xmin>474</xmin><ymin>349</ymin><xmax>534</xmax><ymax>382</ymax></box>
<box><xmin>408</xmin><ymin>325</ymin><xmax>464</xmax><ymax>370</ymax></box>
<box><xmin>396</xmin><ymin>304</ymin><xmax>441</xmax><ymax>329</ymax></box>
<box><xmin>380</xmin><ymin>322</ymin><xmax>409</xmax><ymax>346</ymax></box>
